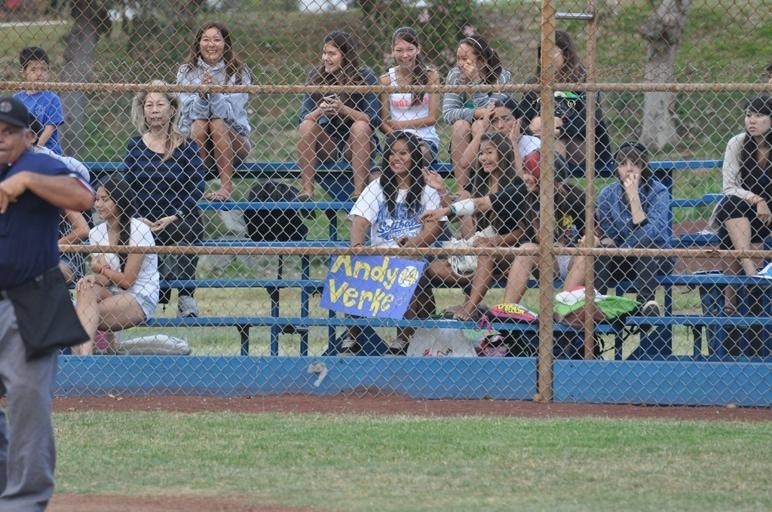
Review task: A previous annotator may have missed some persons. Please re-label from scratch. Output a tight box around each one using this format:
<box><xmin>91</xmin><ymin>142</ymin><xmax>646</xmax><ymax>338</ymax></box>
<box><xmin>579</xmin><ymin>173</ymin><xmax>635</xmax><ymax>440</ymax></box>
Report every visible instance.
<box><xmin>175</xmin><ymin>21</ymin><xmax>253</xmax><ymax>202</ymax></box>
<box><xmin>709</xmin><ymin>97</ymin><xmax>772</xmax><ymax>314</ymax></box>
<box><xmin>72</xmin><ymin>181</ymin><xmax>159</xmax><ymax>355</ymax></box>
<box><xmin>124</xmin><ymin>79</ymin><xmax>205</xmax><ymax>316</ymax></box>
<box><xmin>13</xmin><ymin>47</ymin><xmax>64</xmax><ymax>154</ymax></box>
<box><xmin>57</xmin><ymin>202</ymin><xmax>91</xmax><ymax>288</ymax></box>
<box><xmin>0</xmin><ymin>97</ymin><xmax>95</xmax><ymax>511</ymax></box>
<box><xmin>295</xmin><ymin>24</ymin><xmax>671</xmax><ymax>318</ymax></box>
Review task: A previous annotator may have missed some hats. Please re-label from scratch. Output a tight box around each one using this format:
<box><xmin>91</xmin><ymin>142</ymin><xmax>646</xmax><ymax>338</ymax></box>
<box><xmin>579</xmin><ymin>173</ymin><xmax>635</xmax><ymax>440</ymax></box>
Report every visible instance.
<box><xmin>0</xmin><ymin>96</ymin><xmax>31</xmax><ymax>129</ymax></box>
<box><xmin>613</xmin><ymin>141</ymin><xmax>648</xmax><ymax>160</ymax></box>
<box><xmin>524</xmin><ymin>149</ymin><xmax>569</xmax><ymax>184</ymax></box>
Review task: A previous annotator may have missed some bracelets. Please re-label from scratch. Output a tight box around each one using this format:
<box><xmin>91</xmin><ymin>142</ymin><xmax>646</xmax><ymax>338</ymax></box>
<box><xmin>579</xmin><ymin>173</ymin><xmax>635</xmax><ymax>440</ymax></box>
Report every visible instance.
<box><xmin>174</xmin><ymin>210</ymin><xmax>185</xmax><ymax>220</ymax></box>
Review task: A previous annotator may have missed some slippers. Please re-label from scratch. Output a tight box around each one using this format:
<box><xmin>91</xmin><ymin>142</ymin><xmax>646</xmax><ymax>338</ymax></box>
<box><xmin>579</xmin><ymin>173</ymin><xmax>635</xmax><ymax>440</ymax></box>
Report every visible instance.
<box><xmin>298</xmin><ymin>194</ymin><xmax>316</xmax><ymax>219</ymax></box>
<box><xmin>207</xmin><ymin>191</ymin><xmax>231</xmax><ymax>202</ymax></box>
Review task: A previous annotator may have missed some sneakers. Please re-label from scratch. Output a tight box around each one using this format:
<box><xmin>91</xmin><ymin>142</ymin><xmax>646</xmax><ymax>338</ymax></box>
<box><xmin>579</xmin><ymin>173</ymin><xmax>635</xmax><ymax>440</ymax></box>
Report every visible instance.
<box><xmin>385</xmin><ymin>337</ymin><xmax>408</xmax><ymax>356</ymax></box>
<box><xmin>337</xmin><ymin>336</ymin><xmax>361</xmax><ymax>356</ymax></box>
<box><xmin>177</xmin><ymin>295</ymin><xmax>199</xmax><ymax>317</ymax></box>
<box><xmin>640</xmin><ymin>300</ymin><xmax>659</xmax><ymax>333</ymax></box>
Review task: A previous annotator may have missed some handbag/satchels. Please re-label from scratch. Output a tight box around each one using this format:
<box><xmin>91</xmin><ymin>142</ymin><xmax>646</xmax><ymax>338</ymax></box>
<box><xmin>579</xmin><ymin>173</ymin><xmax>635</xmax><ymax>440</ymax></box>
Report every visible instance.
<box><xmin>243</xmin><ymin>182</ymin><xmax>309</xmax><ymax>241</ymax></box>
<box><xmin>553</xmin><ymin>295</ymin><xmax>642</xmax><ymax>329</ymax></box>
<box><xmin>406</xmin><ymin>325</ymin><xmax>478</xmax><ymax>358</ymax></box>
<box><xmin>442</xmin><ymin>225</ymin><xmax>501</xmax><ymax>277</ymax></box>
<box><xmin>553</xmin><ymin>91</ymin><xmax>578</xmax><ymax>121</ymax></box>
